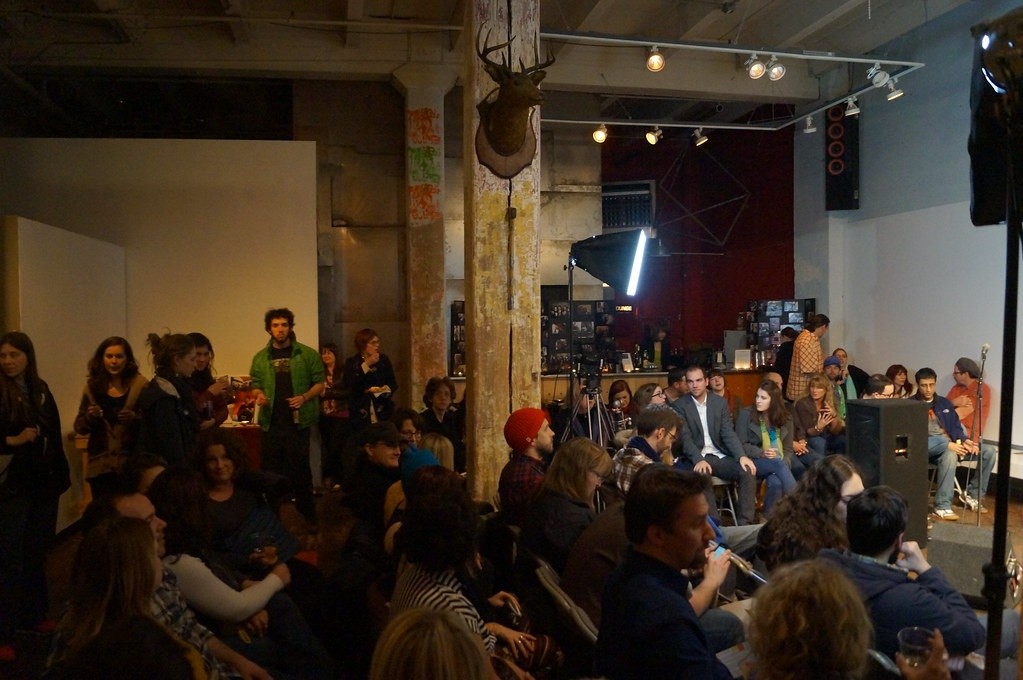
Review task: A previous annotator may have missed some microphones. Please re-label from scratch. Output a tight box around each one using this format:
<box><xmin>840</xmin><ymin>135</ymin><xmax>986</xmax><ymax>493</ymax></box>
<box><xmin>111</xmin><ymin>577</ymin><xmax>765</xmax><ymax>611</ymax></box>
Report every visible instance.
<box><xmin>982</xmin><ymin>343</ymin><xmax>990</xmax><ymax>354</ymax></box>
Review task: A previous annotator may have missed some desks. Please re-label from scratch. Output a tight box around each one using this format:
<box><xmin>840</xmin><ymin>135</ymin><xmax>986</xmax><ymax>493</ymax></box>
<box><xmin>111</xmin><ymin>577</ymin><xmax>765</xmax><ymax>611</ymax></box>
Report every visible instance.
<box><xmin>217</xmin><ymin>412</ymin><xmax>262</xmax><ymax>471</ymax></box>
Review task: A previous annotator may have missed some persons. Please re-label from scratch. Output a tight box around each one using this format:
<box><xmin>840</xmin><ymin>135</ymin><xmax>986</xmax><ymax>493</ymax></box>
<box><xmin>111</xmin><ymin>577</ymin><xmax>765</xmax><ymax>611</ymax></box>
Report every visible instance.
<box><xmin>786</xmin><ymin>314</ymin><xmax>830</xmax><ymax>403</ymax></box>
<box><xmin>662</xmin><ymin>367</ymin><xmax>689</xmax><ymax>404</ymax></box>
<box><xmin>832</xmin><ymin>348</ymin><xmax>869</xmax><ymax>399</ymax></box>
<box><xmin>794</xmin><ymin>373</ymin><xmax>846</xmax><ymax>457</ymax></box>
<box><xmin>606</xmin><ymin>380</ymin><xmax>633</xmax><ymax>414</ymax></box>
<box><xmin>764</xmin><ymin>371</ymin><xmax>823</xmax><ymax>481</ymax></box>
<box><xmin>640</xmin><ymin>322</ymin><xmax>671</xmax><ymax>368</ymax></box>
<box><xmin>859</xmin><ymin>374</ymin><xmax>895</xmax><ymax>399</ymax></box>
<box><xmin>885</xmin><ymin>364</ymin><xmax>914</xmax><ymax>399</ymax></box>
<box><xmin>707</xmin><ymin>369</ymin><xmax>734</xmax><ymax>422</ymax></box>
<box><xmin>944</xmin><ymin>358</ymin><xmax>991</xmax><ymax>443</ymax></box>
<box><xmin>906</xmin><ymin>368</ymin><xmax>996</xmax><ymax>520</ymax></box>
<box><xmin>735</xmin><ymin>380</ymin><xmax>798</xmax><ymax>512</ymax></box>
<box><xmin>0</xmin><ymin>307</ymin><xmax>1022</xmax><ymax>680</ymax></box>
<box><xmin>670</xmin><ymin>364</ymin><xmax>757</xmax><ymax>526</ymax></box>
<box><xmin>823</xmin><ymin>356</ymin><xmax>846</xmax><ymax>427</ymax></box>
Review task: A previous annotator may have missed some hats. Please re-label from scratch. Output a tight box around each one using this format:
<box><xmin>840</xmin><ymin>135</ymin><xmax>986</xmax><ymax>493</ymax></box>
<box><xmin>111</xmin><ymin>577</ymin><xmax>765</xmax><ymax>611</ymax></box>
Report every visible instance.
<box><xmin>956</xmin><ymin>358</ymin><xmax>980</xmax><ymax>379</ymax></box>
<box><xmin>823</xmin><ymin>356</ymin><xmax>840</xmax><ymax>368</ymax></box>
<box><xmin>398</xmin><ymin>445</ymin><xmax>443</xmax><ymax>490</ymax></box>
<box><xmin>361</xmin><ymin>421</ymin><xmax>409</xmax><ymax>443</ymax></box>
<box><xmin>503</xmin><ymin>407</ymin><xmax>545</xmax><ymax>453</ymax></box>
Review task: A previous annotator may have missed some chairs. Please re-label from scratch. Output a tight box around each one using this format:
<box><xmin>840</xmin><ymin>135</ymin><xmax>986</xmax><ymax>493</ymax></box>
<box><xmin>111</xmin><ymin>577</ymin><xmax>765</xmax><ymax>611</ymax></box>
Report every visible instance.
<box><xmin>528</xmin><ymin>544</ymin><xmax>599</xmax><ymax>642</ymax></box>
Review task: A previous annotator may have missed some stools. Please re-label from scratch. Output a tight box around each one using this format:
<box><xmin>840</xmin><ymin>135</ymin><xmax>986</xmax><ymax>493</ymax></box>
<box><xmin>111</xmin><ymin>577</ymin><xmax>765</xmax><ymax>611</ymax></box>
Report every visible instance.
<box><xmin>928</xmin><ymin>461</ymin><xmax>962</xmax><ymax>497</ymax></box>
<box><xmin>710</xmin><ymin>476</ymin><xmax>739</xmax><ymax>526</ymax></box>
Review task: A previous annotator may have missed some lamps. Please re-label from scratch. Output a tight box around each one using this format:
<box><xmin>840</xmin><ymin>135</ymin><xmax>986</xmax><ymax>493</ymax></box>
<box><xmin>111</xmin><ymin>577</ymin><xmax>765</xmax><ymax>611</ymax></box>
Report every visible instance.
<box><xmin>645</xmin><ymin>45</ymin><xmax>666</xmax><ymax>73</ymax></box>
<box><xmin>803</xmin><ymin>117</ymin><xmax>816</xmax><ymax>134</ymax></box>
<box><xmin>886</xmin><ymin>78</ymin><xmax>904</xmax><ymax>101</ymax></box>
<box><xmin>865</xmin><ymin>63</ymin><xmax>889</xmax><ymax>88</ymax></box>
<box><xmin>646</xmin><ymin>126</ymin><xmax>663</xmax><ymax>145</ymax></box>
<box><xmin>592</xmin><ymin>124</ymin><xmax>608</xmax><ymax>143</ymax></box>
<box><xmin>745</xmin><ymin>54</ymin><xmax>786</xmax><ymax>81</ymax></box>
<box><xmin>844</xmin><ymin>96</ymin><xmax>860</xmax><ymax>116</ymax></box>
<box><xmin>694</xmin><ymin>127</ymin><xmax>708</xmax><ymax>146</ymax></box>
<box><xmin>563</xmin><ymin>228</ymin><xmax>646</xmax><ymax>438</ymax></box>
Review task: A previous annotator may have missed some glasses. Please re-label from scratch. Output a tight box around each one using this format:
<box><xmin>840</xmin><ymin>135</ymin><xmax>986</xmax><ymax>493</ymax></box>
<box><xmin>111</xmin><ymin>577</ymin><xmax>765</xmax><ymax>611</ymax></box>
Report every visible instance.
<box><xmin>651</xmin><ymin>391</ymin><xmax>662</xmax><ymax>399</ymax></box>
<box><xmin>657</xmin><ymin>427</ymin><xmax>678</xmax><ymax>442</ymax></box>
<box><xmin>397</xmin><ymin>429</ymin><xmax>422</xmax><ymax>438</ymax></box>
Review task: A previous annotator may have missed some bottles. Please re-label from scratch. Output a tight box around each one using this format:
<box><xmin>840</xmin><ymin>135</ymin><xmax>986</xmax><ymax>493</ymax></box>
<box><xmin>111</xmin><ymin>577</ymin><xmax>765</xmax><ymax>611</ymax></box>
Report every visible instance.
<box><xmin>750</xmin><ymin>344</ymin><xmax>779</xmax><ymax>370</ymax></box>
<box><xmin>631</xmin><ymin>344</ymin><xmax>649</xmax><ymax>370</ymax></box>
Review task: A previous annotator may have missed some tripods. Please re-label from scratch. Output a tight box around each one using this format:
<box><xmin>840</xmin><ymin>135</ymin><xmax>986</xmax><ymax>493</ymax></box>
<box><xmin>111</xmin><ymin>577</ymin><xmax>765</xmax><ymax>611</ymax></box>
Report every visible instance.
<box><xmin>560</xmin><ymin>365</ymin><xmax>617</xmax><ymax>514</ymax></box>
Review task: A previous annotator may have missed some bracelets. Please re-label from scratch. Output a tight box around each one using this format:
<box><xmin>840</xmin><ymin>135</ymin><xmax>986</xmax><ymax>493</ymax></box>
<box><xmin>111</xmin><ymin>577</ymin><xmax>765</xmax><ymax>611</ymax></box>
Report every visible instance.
<box><xmin>815</xmin><ymin>426</ymin><xmax>823</xmax><ymax>433</ymax></box>
<box><xmin>299</xmin><ymin>394</ymin><xmax>305</xmax><ymax>401</ymax></box>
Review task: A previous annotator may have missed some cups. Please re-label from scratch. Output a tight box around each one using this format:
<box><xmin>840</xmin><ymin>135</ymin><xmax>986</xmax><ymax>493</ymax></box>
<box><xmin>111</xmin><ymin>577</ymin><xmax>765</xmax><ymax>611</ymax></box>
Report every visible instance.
<box><xmin>201</xmin><ymin>400</ymin><xmax>213</xmax><ymax>419</ymax></box>
<box><xmin>898</xmin><ymin>627</ymin><xmax>935</xmax><ymax>671</ymax></box>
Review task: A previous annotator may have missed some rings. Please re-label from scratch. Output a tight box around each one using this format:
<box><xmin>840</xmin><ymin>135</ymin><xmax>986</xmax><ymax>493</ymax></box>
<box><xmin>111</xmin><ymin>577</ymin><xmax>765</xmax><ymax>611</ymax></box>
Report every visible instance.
<box><xmin>518</xmin><ymin>641</ymin><xmax>521</xmax><ymax>645</ymax></box>
<box><xmin>520</xmin><ymin>636</ymin><xmax>523</xmax><ymax>639</ymax></box>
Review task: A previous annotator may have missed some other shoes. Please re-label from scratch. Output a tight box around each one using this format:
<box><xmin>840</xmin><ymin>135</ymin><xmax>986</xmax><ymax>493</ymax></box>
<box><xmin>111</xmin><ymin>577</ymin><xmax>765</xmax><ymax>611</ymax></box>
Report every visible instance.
<box><xmin>959</xmin><ymin>491</ymin><xmax>987</xmax><ymax>513</ymax></box>
<box><xmin>933</xmin><ymin>506</ymin><xmax>959</xmax><ymax>520</ymax></box>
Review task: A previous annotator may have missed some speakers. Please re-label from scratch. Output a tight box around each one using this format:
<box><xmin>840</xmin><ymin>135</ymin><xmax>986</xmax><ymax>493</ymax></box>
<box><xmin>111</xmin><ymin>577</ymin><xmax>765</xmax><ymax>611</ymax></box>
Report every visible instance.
<box><xmin>845</xmin><ymin>397</ymin><xmax>929</xmax><ymax>550</ymax></box>
<box><xmin>924</xmin><ymin>521</ymin><xmax>1023</xmax><ymax>610</ymax></box>
<box><xmin>825</xmin><ymin>101</ymin><xmax>860</xmax><ymax>211</ymax></box>
<box><xmin>968</xmin><ymin>20</ymin><xmax>1023</xmax><ymax>227</ymax></box>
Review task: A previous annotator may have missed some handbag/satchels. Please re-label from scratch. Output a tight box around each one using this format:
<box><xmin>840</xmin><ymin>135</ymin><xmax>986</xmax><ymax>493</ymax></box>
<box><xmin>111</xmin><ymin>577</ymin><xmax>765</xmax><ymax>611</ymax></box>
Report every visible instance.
<box><xmin>323</xmin><ymin>400</ymin><xmax>348</xmax><ymax>419</ymax></box>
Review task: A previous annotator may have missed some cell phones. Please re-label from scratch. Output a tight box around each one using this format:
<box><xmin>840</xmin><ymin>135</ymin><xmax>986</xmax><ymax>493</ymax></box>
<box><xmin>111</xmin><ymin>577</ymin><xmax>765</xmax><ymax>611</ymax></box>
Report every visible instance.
<box><xmin>714</xmin><ymin>542</ymin><xmax>729</xmax><ymax>559</ymax></box>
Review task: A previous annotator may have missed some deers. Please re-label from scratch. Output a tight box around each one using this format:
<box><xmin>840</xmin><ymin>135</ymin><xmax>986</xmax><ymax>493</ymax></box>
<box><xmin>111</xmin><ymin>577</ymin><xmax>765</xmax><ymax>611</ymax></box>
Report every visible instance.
<box><xmin>475</xmin><ymin>19</ymin><xmax>556</xmax><ymax>156</ymax></box>
